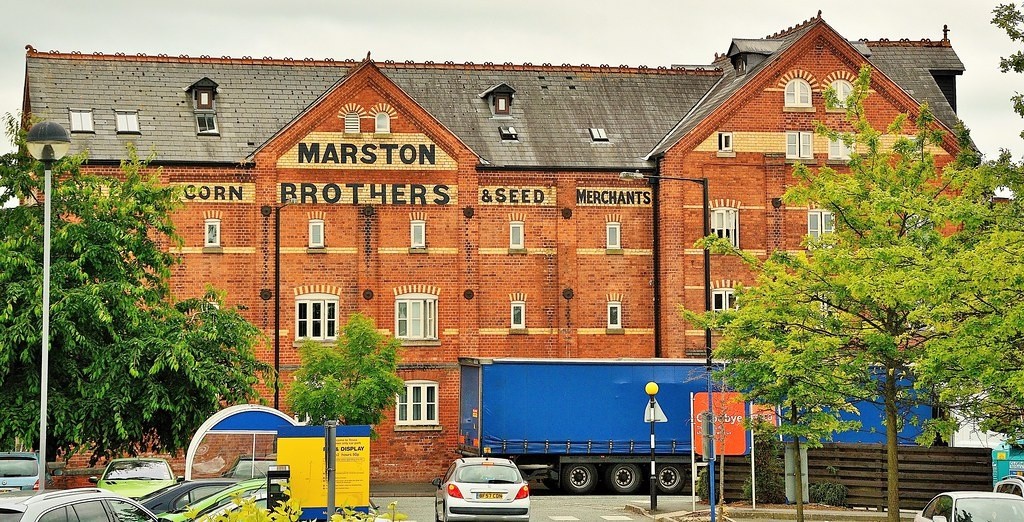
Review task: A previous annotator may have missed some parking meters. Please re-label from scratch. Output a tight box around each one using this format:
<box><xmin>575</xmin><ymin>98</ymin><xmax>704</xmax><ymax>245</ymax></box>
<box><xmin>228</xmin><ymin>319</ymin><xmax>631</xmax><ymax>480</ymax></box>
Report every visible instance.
<box><xmin>266</xmin><ymin>465</ymin><xmax>290</xmax><ymax>521</ymax></box>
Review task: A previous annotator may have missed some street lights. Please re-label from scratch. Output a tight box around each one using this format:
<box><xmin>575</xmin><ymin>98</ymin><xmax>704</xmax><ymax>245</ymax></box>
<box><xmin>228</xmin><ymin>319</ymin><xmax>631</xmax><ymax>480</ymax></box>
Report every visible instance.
<box><xmin>24</xmin><ymin>119</ymin><xmax>71</xmax><ymax>490</ymax></box>
<box><xmin>620</xmin><ymin>170</ymin><xmax>713</xmax><ymax>504</ymax></box>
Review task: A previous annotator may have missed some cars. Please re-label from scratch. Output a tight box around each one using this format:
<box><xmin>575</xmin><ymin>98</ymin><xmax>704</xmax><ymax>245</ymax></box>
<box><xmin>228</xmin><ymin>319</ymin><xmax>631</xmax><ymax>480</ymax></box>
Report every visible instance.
<box><xmin>914</xmin><ymin>475</ymin><xmax>1024</xmax><ymax>522</ymax></box>
<box><xmin>430</xmin><ymin>458</ymin><xmax>530</xmax><ymax>522</ymax></box>
<box><xmin>0</xmin><ymin>451</ymin><xmax>277</xmax><ymax>522</ymax></box>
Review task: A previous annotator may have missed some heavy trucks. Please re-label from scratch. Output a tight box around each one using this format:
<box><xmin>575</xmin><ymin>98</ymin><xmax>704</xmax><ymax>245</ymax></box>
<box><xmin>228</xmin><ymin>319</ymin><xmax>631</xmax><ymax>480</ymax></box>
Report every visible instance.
<box><xmin>457</xmin><ymin>359</ymin><xmax>1012</xmax><ymax>494</ymax></box>
